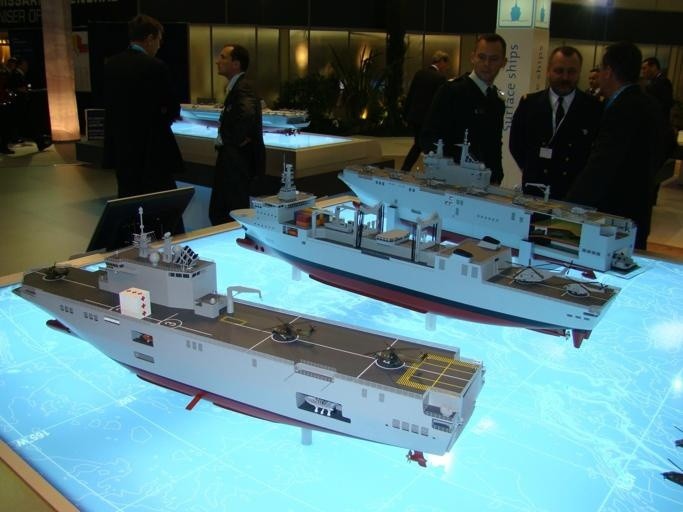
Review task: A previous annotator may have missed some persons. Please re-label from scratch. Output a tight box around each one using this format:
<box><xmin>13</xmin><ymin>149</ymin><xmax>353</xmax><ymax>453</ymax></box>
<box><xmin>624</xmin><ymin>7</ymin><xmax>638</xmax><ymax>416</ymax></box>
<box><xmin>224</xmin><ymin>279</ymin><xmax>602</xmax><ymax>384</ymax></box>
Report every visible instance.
<box><xmin>101</xmin><ymin>12</ymin><xmax>182</xmax><ymax>199</ymax></box>
<box><xmin>401</xmin><ymin>51</ymin><xmax>448</xmax><ymax>174</ymax></box>
<box><xmin>508</xmin><ymin>48</ymin><xmax>600</xmax><ymax>197</ymax></box>
<box><xmin>0</xmin><ymin>57</ymin><xmax>52</xmax><ymax>154</ymax></box>
<box><xmin>637</xmin><ymin>58</ymin><xmax>682</xmax><ymax>184</ymax></box>
<box><xmin>576</xmin><ymin>44</ymin><xmax>673</xmax><ymax>252</ymax></box>
<box><xmin>421</xmin><ymin>33</ymin><xmax>504</xmax><ymax>186</ymax></box>
<box><xmin>207</xmin><ymin>43</ymin><xmax>266</xmax><ymax>226</ymax></box>
<box><xmin>585</xmin><ymin>68</ymin><xmax>604</xmax><ymax>99</ymax></box>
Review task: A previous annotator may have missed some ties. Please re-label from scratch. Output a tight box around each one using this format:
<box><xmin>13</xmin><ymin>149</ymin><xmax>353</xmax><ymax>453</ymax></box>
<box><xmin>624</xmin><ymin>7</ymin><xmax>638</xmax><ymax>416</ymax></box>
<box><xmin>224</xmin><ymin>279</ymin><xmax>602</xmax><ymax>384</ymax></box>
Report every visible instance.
<box><xmin>485</xmin><ymin>85</ymin><xmax>492</xmax><ymax>97</ymax></box>
<box><xmin>554</xmin><ymin>96</ymin><xmax>565</xmax><ymax>128</ymax></box>
